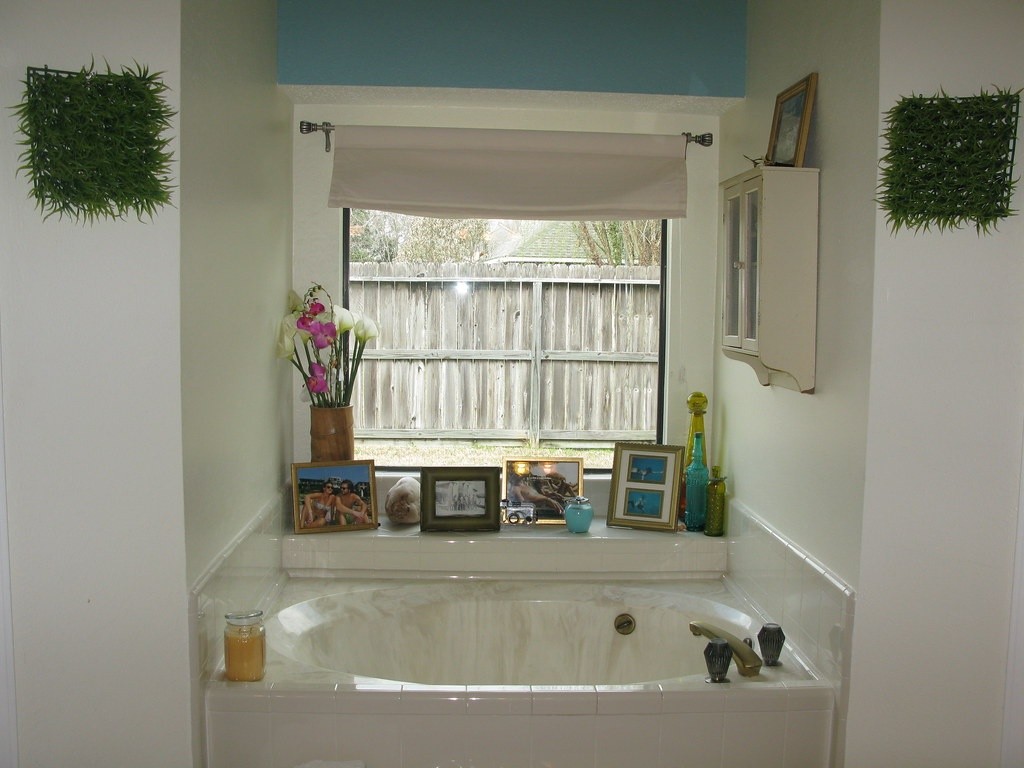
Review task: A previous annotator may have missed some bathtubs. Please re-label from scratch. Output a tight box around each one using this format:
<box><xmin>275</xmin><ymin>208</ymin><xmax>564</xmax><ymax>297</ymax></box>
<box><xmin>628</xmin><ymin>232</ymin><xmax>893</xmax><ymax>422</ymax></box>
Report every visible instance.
<box><xmin>202</xmin><ymin>575</ymin><xmax>837</xmax><ymax>768</ymax></box>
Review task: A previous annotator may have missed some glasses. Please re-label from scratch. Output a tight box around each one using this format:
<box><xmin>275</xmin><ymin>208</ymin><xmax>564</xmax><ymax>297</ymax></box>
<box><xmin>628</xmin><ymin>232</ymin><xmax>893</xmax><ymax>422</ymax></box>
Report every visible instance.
<box><xmin>326</xmin><ymin>486</ymin><xmax>334</xmax><ymax>489</ymax></box>
<box><xmin>340</xmin><ymin>486</ymin><xmax>348</xmax><ymax>490</ymax></box>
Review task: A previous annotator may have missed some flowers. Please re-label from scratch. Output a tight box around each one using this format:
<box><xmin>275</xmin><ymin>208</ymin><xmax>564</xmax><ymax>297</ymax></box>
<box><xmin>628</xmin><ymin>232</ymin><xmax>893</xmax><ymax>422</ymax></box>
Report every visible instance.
<box><xmin>277</xmin><ymin>280</ymin><xmax>377</xmax><ymax>408</ymax></box>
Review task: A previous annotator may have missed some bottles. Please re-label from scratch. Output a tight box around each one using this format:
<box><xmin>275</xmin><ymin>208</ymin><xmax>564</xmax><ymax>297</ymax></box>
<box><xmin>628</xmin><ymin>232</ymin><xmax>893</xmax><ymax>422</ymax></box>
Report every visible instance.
<box><xmin>704</xmin><ymin>465</ymin><xmax>727</xmax><ymax>537</ymax></box>
<box><xmin>686</xmin><ymin>432</ymin><xmax>709</xmax><ymax>532</ymax></box>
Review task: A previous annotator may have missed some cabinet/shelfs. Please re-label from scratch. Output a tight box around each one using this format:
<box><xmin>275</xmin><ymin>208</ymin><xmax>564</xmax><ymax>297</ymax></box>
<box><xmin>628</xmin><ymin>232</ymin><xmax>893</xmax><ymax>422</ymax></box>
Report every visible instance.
<box><xmin>718</xmin><ymin>164</ymin><xmax>820</xmax><ymax>395</ymax></box>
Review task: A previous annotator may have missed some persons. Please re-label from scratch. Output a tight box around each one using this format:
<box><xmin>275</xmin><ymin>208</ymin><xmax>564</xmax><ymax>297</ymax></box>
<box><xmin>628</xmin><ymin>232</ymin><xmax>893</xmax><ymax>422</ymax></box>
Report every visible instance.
<box><xmin>299</xmin><ymin>479</ymin><xmax>372</xmax><ymax>528</ymax></box>
<box><xmin>448</xmin><ymin>494</ymin><xmax>478</xmax><ymax>511</ymax></box>
<box><xmin>509</xmin><ymin>466</ymin><xmax>576</xmax><ymax>515</ymax></box>
<box><xmin>638</xmin><ymin>466</ymin><xmax>652</xmax><ymax>481</ymax></box>
<box><xmin>628</xmin><ymin>496</ymin><xmax>648</xmax><ymax>513</ymax></box>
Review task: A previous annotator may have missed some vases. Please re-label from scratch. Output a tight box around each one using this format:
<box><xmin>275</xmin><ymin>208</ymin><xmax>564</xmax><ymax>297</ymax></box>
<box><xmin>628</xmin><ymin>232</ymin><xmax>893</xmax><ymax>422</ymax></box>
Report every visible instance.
<box><xmin>309</xmin><ymin>403</ymin><xmax>355</xmax><ymax>462</ymax></box>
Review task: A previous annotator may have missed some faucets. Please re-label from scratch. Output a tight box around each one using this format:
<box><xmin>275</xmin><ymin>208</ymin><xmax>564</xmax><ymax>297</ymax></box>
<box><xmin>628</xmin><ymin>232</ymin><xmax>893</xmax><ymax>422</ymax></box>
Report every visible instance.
<box><xmin>688</xmin><ymin>619</ymin><xmax>764</xmax><ymax>679</ymax></box>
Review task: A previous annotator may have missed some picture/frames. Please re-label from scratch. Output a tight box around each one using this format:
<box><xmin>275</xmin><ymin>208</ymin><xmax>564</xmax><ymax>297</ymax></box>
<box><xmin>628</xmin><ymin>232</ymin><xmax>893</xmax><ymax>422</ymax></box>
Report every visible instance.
<box><xmin>290</xmin><ymin>459</ymin><xmax>379</xmax><ymax>534</ymax></box>
<box><xmin>606</xmin><ymin>443</ymin><xmax>685</xmax><ymax>534</ymax></box>
<box><xmin>420</xmin><ymin>467</ymin><xmax>502</xmax><ymax>533</ymax></box>
<box><xmin>502</xmin><ymin>456</ymin><xmax>583</xmax><ymax>525</ymax></box>
<box><xmin>765</xmin><ymin>72</ymin><xmax>818</xmax><ymax>168</ymax></box>
